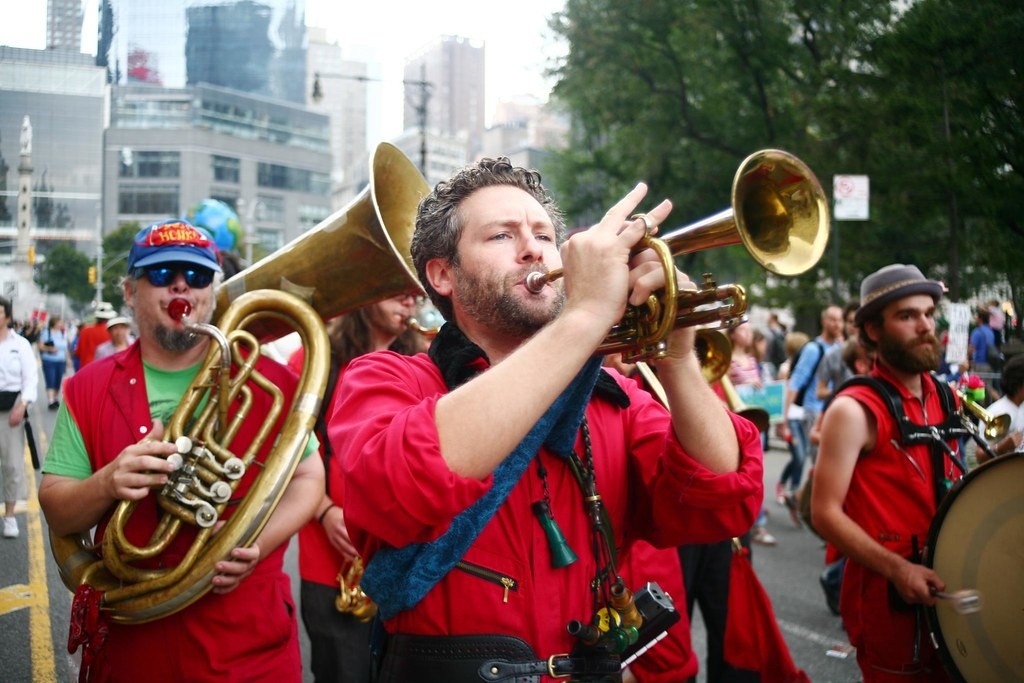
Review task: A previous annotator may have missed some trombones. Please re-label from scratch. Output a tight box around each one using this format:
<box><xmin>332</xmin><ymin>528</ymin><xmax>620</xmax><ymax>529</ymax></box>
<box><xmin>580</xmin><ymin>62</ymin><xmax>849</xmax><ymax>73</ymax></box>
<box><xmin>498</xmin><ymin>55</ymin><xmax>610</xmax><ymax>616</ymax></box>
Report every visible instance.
<box><xmin>956</xmin><ymin>389</ymin><xmax>1012</xmax><ymax>442</ymax></box>
<box><xmin>636</xmin><ymin>329</ymin><xmax>743</xmax><ymax>552</ymax></box>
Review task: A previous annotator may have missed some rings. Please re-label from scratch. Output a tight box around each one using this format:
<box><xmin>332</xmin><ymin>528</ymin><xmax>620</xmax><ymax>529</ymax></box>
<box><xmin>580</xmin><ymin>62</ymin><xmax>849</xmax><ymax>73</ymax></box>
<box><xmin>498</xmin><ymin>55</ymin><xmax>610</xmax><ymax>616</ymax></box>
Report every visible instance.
<box><xmin>639</xmin><ymin>216</ymin><xmax>651</xmax><ymax>240</ymax></box>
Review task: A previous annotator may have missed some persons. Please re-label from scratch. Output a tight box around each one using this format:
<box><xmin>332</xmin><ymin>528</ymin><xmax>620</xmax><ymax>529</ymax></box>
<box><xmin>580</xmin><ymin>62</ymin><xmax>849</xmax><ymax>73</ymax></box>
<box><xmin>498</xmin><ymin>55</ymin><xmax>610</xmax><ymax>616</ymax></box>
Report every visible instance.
<box><xmin>39</xmin><ymin>215</ymin><xmax>326</xmax><ymax>683</ymax></box>
<box><xmin>938</xmin><ymin>301</ymin><xmax>1024</xmax><ymax>470</ymax></box>
<box><xmin>811</xmin><ymin>264</ymin><xmax>967</xmax><ymax>683</ymax></box>
<box><xmin>769</xmin><ymin>302</ymin><xmax>859</xmax><ymax>526</ymax></box>
<box><xmin>0</xmin><ymin>296</ymin><xmax>38</xmax><ymax>538</ymax></box>
<box><xmin>299</xmin><ymin>155</ymin><xmax>762</xmax><ymax>683</ymax></box>
<box><xmin>10</xmin><ymin>316</ymin><xmax>73</xmax><ymax>409</ymax></box>
<box><xmin>73</xmin><ymin>300</ymin><xmax>136</xmax><ymax>373</ymax></box>
<box><xmin>723</xmin><ymin>315</ymin><xmax>776</xmax><ymax>546</ymax></box>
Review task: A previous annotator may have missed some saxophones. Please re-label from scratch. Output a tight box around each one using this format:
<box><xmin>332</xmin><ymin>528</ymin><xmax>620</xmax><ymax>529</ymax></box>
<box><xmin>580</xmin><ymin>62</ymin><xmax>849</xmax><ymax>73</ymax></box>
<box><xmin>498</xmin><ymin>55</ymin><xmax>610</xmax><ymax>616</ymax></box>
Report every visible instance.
<box><xmin>335</xmin><ymin>314</ymin><xmax>442</xmax><ymax>624</ymax></box>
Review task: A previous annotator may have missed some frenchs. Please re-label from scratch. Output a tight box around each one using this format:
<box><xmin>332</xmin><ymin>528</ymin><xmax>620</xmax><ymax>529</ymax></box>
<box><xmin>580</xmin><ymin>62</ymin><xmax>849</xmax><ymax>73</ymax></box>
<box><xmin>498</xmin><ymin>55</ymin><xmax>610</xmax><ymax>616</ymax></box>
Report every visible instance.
<box><xmin>47</xmin><ymin>141</ymin><xmax>432</xmax><ymax>625</ymax></box>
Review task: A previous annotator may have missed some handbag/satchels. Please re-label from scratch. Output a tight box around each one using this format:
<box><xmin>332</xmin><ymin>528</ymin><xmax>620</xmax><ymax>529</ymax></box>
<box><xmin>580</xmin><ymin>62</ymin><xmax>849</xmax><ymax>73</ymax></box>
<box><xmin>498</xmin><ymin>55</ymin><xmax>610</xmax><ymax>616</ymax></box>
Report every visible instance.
<box><xmin>44</xmin><ymin>326</ymin><xmax>54</xmax><ymax>347</ymax></box>
<box><xmin>986</xmin><ymin>346</ymin><xmax>1005</xmax><ymax>369</ymax></box>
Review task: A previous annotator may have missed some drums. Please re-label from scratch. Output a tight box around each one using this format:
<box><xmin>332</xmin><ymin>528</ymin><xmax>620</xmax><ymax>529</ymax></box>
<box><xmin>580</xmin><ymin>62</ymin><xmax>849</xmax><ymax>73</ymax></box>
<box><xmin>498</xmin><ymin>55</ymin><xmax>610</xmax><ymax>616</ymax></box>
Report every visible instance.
<box><xmin>924</xmin><ymin>451</ymin><xmax>1024</xmax><ymax>683</ymax></box>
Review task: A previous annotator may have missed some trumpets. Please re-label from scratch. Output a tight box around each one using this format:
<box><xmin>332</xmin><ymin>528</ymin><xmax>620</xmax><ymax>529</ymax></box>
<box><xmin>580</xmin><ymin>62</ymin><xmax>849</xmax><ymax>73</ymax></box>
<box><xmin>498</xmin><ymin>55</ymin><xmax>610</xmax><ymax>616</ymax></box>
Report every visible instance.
<box><xmin>719</xmin><ymin>372</ymin><xmax>770</xmax><ymax>433</ymax></box>
<box><xmin>525</xmin><ymin>148</ymin><xmax>830</xmax><ymax>365</ymax></box>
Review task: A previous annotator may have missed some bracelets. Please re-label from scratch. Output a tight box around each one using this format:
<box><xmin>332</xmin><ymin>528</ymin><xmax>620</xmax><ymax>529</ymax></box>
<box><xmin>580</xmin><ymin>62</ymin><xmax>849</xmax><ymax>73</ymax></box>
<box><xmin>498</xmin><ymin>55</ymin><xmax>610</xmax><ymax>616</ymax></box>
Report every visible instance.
<box><xmin>995</xmin><ymin>443</ymin><xmax>1001</xmax><ymax>455</ymax></box>
<box><xmin>20</xmin><ymin>400</ymin><xmax>27</xmax><ymax>407</ymax></box>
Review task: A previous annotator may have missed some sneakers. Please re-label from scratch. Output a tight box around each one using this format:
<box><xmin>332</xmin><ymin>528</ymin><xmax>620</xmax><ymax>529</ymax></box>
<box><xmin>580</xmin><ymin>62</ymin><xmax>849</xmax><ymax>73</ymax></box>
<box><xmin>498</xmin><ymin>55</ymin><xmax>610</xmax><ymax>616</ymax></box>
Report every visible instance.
<box><xmin>3</xmin><ymin>516</ymin><xmax>19</xmax><ymax>538</ymax></box>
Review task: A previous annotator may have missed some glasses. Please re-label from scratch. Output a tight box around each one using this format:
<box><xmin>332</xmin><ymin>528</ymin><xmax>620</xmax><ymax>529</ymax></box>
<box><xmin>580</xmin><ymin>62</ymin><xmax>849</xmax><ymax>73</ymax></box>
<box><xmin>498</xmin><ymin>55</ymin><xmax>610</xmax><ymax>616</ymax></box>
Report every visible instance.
<box><xmin>133</xmin><ymin>260</ymin><xmax>213</xmax><ymax>288</ymax></box>
<box><xmin>395</xmin><ymin>292</ymin><xmax>426</xmax><ymax>307</ymax></box>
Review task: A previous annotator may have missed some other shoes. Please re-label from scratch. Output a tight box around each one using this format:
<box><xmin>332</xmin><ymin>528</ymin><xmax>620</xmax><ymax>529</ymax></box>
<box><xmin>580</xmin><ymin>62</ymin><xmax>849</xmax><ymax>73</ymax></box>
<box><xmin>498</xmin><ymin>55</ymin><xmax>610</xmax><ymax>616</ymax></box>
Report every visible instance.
<box><xmin>788</xmin><ymin>499</ymin><xmax>802</xmax><ymax>527</ymax></box>
<box><xmin>54</xmin><ymin>401</ymin><xmax>58</xmax><ymax>406</ymax></box>
<box><xmin>822</xmin><ymin>559</ymin><xmax>846</xmax><ymax>613</ymax></box>
<box><xmin>751</xmin><ymin>525</ymin><xmax>777</xmax><ymax>546</ymax></box>
<box><xmin>776</xmin><ymin>482</ymin><xmax>786</xmax><ymax>504</ymax></box>
<box><xmin>49</xmin><ymin>404</ymin><xmax>56</xmax><ymax>410</ymax></box>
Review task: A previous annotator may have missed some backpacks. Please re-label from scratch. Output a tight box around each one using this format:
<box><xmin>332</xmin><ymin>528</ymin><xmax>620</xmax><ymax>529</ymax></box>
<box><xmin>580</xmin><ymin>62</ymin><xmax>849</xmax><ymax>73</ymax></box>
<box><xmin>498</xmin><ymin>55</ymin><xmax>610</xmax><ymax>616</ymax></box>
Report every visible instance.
<box><xmin>789</xmin><ymin>339</ymin><xmax>825</xmax><ymax>406</ymax></box>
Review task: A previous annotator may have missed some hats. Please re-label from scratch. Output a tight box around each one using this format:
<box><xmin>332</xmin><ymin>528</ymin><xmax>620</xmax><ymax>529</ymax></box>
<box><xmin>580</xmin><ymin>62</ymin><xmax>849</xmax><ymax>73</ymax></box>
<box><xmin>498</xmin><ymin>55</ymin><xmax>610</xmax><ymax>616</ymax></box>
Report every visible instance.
<box><xmin>95</xmin><ymin>302</ymin><xmax>118</xmax><ymax>319</ymax></box>
<box><xmin>854</xmin><ymin>264</ymin><xmax>942</xmax><ymax>323</ymax></box>
<box><xmin>105</xmin><ymin>318</ymin><xmax>130</xmax><ymax>329</ymax></box>
<box><xmin>126</xmin><ymin>219</ymin><xmax>223</xmax><ymax>272</ymax></box>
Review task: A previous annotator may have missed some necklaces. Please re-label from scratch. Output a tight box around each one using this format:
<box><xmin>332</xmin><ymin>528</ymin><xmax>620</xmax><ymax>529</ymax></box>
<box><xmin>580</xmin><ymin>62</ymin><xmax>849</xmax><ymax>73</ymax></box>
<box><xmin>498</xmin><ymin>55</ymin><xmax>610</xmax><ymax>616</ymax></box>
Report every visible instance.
<box><xmin>555</xmin><ymin>415</ymin><xmax>642</xmax><ymax>652</ymax></box>
